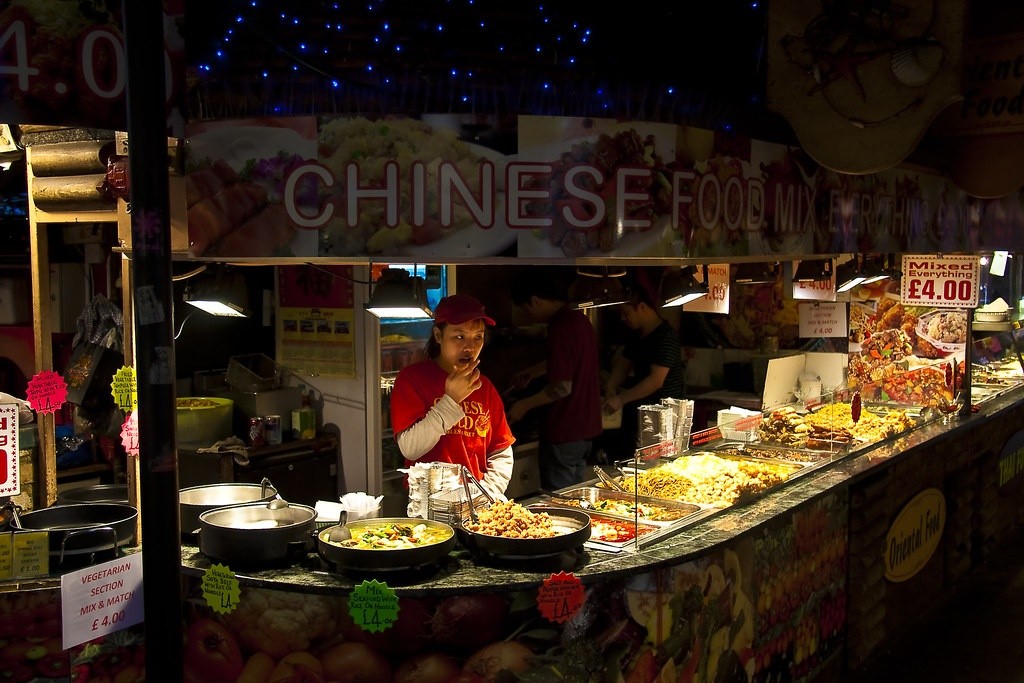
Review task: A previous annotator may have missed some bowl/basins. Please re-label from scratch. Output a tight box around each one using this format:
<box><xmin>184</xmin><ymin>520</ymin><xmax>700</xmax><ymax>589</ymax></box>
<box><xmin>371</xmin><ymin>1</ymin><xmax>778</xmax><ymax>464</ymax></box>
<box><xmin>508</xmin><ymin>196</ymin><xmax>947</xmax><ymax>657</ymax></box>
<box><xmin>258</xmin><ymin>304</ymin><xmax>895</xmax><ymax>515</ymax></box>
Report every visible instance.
<box><xmin>176</xmin><ymin>397</ymin><xmax>234</xmax><ymax>444</ymax></box>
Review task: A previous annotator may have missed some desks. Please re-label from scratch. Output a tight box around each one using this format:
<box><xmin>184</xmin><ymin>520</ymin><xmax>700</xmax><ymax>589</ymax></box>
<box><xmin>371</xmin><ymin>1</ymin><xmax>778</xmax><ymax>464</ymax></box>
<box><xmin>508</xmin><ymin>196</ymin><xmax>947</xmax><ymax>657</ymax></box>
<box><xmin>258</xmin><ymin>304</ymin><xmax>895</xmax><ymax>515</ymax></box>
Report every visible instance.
<box><xmin>695</xmin><ymin>390</ymin><xmax>761</xmax><ymax>430</ymax></box>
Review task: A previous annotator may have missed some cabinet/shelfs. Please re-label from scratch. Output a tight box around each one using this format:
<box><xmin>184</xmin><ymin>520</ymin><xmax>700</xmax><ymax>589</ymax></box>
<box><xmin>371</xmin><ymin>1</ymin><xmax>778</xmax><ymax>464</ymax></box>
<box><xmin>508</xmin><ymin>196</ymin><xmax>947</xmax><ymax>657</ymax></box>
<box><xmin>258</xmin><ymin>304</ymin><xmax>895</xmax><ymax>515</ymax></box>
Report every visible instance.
<box><xmin>179</xmin><ymin>434</ymin><xmax>339</xmax><ymax>508</ymax></box>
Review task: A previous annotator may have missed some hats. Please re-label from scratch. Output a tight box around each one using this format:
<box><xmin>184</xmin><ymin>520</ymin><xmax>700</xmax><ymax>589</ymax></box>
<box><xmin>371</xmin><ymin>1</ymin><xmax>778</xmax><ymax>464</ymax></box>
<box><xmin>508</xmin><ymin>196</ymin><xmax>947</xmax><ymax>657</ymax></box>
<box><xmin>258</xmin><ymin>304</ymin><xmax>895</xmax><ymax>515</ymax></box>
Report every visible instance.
<box><xmin>434</xmin><ymin>294</ymin><xmax>495</xmax><ymax>327</ymax></box>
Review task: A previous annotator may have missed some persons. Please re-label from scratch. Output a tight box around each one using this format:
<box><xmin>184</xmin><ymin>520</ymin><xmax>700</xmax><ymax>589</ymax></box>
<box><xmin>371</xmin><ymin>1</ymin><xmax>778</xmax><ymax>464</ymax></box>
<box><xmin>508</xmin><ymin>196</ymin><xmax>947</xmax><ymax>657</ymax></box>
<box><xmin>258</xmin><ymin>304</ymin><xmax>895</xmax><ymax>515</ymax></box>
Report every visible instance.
<box><xmin>390</xmin><ymin>294</ymin><xmax>516</xmax><ymax>515</ymax></box>
<box><xmin>508</xmin><ymin>287</ymin><xmax>603</xmax><ymax>496</ymax></box>
<box><xmin>601</xmin><ymin>292</ymin><xmax>686</xmax><ymax>465</ymax></box>
<box><xmin>0</xmin><ymin>356</ymin><xmax>38</xmax><ymax>423</ymax></box>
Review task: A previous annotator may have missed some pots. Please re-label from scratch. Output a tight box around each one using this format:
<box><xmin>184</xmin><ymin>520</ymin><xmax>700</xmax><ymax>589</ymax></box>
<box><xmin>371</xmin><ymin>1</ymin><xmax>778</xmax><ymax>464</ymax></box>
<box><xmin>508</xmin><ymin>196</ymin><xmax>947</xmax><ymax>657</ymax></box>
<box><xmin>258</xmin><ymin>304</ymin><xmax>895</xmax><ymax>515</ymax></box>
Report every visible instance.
<box><xmin>9</xmin><ymin>503</ymin><xmax>139</xmax><ymax>570</ymax></box>
<box><xmin>198</xmin><ymin>501</ymin><xmax>318</xmax><ymax>564</ymax></box>
<box><xmin>179</xmin><ymin>483</ymin><xmax>277</xmax><ymax>538</ymax></box>
<box><xmin>453</xmin><ymin>502</ymin><xmax>592</xmax><ymax>562</ymax></box>
<box><xmin>317</xmin><ymin>518</ymin><xmax>455</xmax><ymax>576</ymax></box>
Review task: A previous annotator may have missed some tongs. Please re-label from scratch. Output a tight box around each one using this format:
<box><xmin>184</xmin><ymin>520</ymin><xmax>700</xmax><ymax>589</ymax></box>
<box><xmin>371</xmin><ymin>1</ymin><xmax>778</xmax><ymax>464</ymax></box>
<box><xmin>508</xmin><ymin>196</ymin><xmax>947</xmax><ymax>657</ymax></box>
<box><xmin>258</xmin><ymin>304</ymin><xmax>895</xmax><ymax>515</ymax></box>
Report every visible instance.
<box><xmin>461</xmin><ymin>465</ymin><xmax>496</xmax><ymax>525</ymax></box>
<box><xmin>260</xmin><ymin>478</ymin><xmax>283</xmax><ymax>500</ymax></box>
<box><xmin>592</xmin><ymin>465</ymin><xmax>626</xmax><ymax>492</ymax></box>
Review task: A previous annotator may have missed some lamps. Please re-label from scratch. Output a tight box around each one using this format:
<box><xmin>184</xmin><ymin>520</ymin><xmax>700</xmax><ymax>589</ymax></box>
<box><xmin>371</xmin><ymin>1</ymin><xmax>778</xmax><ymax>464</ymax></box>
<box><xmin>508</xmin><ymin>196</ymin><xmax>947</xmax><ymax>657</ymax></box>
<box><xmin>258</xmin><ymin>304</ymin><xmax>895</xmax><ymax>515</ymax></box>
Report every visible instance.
<box><xmin>572</xmin><ymin>270</ymin><xmax>629</xmax><ymax>309</ymax></box>
<box><xmin>361</xmin><ymin>272</ymin><xmax>429</xmax><ymax>321</ymax></box>
<box><xmin>862</xmin><ymin>254</ymin><xmax>891</xmax><ymax>284</ymax></box>
<box><xmin>650</xmin><ymin>266</ymin><xmax>709</xmax><ymax>309</ymax></box>
<box><xmin>733</xmin><ymin>264</ymin><xmax>778</xmax><ymax>284</ymax></box>
<box><xmin>181</xmin><ymin>264</ymin><xmax>251</xmax><ymax>318</ymax></box>
<box><xmin>791</xmin><ymin>260</ymin><xmax>833</xmax><ymax>282</ymax></box>
<box><xmin>836</xmin><ymin>256</ymin><xmax>864</xmax><ymax>292</ymax></box>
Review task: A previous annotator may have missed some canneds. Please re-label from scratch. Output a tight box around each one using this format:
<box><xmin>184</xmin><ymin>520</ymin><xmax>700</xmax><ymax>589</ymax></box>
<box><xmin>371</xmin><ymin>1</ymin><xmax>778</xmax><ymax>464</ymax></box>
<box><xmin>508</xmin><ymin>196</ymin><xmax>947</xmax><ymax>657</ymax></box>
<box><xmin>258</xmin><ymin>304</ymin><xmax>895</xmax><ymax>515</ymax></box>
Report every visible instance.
<box><xmin>249</xmin><ymin>415</ymin><xmax>282</xmax><ymax>448</ymax></box>
